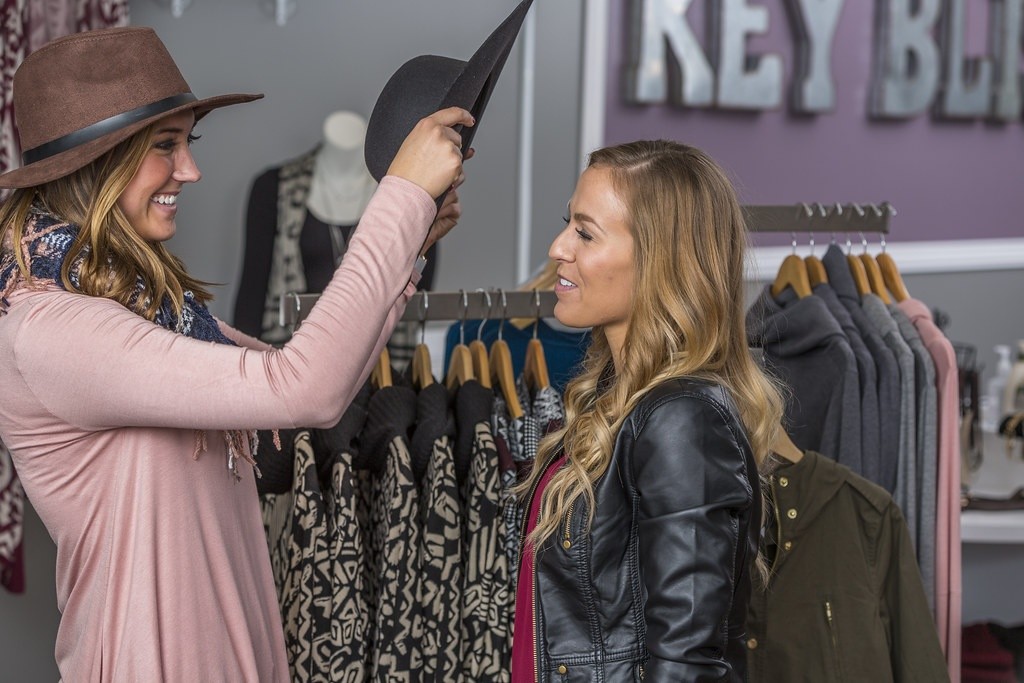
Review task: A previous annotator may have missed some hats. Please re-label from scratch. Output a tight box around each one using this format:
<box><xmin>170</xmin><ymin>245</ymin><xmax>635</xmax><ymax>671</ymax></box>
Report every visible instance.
<box><xmin>365</xmin><ymin>0</ymin><xmax>535</xmax><ymax>291</ymax></box>
<box><xmin>0</xmin><ymin>27</ymin><xmax>264</xmax><ymax>190</ymax></box>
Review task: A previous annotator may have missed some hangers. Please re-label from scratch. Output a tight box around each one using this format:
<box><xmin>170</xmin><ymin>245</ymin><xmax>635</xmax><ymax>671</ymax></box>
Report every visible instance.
<box><xmin>771</xmin><ymin>200</ymin><xmax>910</xmax><ymax>304</ymax></box>
<box><xmin>284</xmin><ymin>285</ymin><xmax>549</xmax><ymax>418</ymax></box>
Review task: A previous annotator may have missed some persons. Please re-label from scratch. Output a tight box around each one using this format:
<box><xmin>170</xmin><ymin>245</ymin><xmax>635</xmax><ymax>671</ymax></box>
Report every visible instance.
<box><xmin>233</xmin><ymin>113</ymin><xmax>438</xmax><ymax>341</ymax></box>
<box><xmin>511</xmin><ymin>138</ymin><xmax>786</xmax><ymax>683</ymax></box>
<box><xmin>0</xmin><ymin>27</ymin><xmax>476</xmax><ymax>682</ymax></box>
